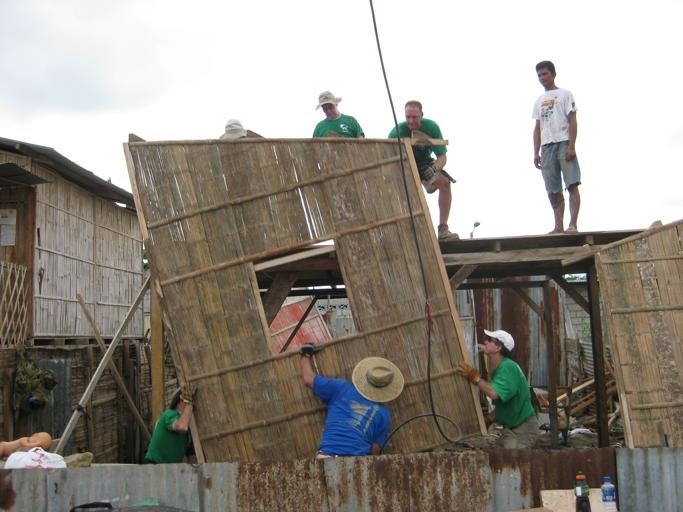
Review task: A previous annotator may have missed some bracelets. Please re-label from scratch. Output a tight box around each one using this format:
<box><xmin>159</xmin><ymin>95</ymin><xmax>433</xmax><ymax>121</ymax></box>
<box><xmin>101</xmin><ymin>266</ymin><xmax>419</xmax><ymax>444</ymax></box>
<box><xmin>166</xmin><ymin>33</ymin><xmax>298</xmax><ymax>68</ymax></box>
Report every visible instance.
<box><xmin>475</xmin><ymin>377</ymin><xmax>482</xmax><ymax>385</ymax></box>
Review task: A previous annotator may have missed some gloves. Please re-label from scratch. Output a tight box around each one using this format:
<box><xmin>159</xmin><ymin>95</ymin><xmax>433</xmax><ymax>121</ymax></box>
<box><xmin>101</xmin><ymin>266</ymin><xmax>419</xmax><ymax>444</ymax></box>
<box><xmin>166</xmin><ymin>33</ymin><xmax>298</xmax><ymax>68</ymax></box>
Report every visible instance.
<box><xmin>301</xmin><ymin>344</ymin><xmax>314</xmax><ymax>356</ymax></box>
<box><xmin>457</xmin><ymin>361</ymin><xmax>479</xmax><ymax>383</ymax></box>
<box><xmin>180</xmin><ymin>382</ymin><xmax>197</xmax><ymax>402</ymax></box>
<box><xmin>421</xmin><ymin>164</ymin><xmax>434</xmax><ymax>180</ymax></box>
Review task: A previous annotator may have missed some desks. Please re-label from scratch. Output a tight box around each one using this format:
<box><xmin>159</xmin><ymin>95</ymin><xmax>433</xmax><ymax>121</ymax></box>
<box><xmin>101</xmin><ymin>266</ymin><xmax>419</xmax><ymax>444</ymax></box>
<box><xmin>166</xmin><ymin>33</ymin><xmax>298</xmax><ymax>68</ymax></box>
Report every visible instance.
<box><xmin>539</xmin><ymin>488</ymin><xmax>618</xmax><ymax>511</ymax></box>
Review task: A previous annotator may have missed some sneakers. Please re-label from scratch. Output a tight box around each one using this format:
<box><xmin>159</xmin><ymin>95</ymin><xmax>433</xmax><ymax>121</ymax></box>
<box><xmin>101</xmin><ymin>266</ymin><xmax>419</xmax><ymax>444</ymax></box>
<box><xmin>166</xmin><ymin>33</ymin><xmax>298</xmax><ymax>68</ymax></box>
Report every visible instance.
<box><xmin>546</xmin><ymin>226</ymin><xmax>578</xmax><ymax>234</ymax></box>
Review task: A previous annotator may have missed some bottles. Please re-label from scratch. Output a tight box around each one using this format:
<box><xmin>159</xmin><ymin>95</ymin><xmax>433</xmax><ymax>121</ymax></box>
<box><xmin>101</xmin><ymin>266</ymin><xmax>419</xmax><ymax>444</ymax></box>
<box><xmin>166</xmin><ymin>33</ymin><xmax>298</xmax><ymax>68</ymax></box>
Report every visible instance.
<box><xmin>574</xmin><ymin>470</ymin><xmax>591</xmax><ymax>512</ymax></box>
<box><xmin>601</xmin><ymin>477</ymin><xmax>618</xmax><ymax>511</ymax></box>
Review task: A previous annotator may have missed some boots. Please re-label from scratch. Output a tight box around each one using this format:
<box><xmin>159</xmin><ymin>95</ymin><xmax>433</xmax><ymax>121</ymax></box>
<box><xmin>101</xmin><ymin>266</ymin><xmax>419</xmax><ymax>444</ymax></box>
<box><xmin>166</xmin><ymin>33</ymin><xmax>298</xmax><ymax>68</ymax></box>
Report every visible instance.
<box><xmin>438</xmin><ymin>224</ymin><xmax>459</xmax><ymax>241</ymax></box>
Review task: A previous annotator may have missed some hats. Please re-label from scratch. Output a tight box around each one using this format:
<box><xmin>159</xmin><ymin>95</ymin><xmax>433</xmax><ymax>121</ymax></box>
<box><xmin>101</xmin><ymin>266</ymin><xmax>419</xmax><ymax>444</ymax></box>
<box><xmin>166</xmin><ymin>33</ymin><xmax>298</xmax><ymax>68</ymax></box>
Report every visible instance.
<box><xmin>484</xmin><ymin>329</ymin><xmax>514</xmax><ymax>351</ymax></box>
<box><xmin>220</xmin><ymin>120</ymin><xmax>247</xmax><ymax>139</ymax></box>
<box><xmin>352</xmin><ymin>357</ymin><xmax>404</xmax><ymax>402</ymax></box>
<box><xmin>316</xmin><ymin>92</ymin><xmax>341</xmax><ymax>110</ymax></box>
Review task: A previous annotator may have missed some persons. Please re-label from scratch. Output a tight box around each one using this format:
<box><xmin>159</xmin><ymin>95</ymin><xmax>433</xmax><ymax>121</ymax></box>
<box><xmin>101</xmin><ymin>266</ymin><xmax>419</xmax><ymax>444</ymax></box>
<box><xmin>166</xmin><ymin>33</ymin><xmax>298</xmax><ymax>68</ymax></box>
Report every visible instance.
<box><xmin>388</xmin><ymin>101</ymin><xmax>459</xmax><ymax>242</ymax></box>
<box><xmin>144</xmin><ymin>382</ymin><xmax>197</xmax><ymax>464</ymax></box>
<box><xmin>457</xmin><ymin>328</ymin><xmax>539</xmax><ymax>449</ymax></box>
<box><xmin>312</xmin><ymin>92</ymin><xmax>364</xmax><ymax>138</ymax></box>
<box><xmin>219</xmin><ymin>119</ymin><xmax>263</xmax><ymax>139</ymax></box>
<box><xmin>531</xmin><ymin>61</ymin><xmax>582</xmax><ymax>234</ymax></box>
<box><xmin>300</xmin><ymin>343</ymin><xmax>404</xmax><ymax>458</ymax></box>
